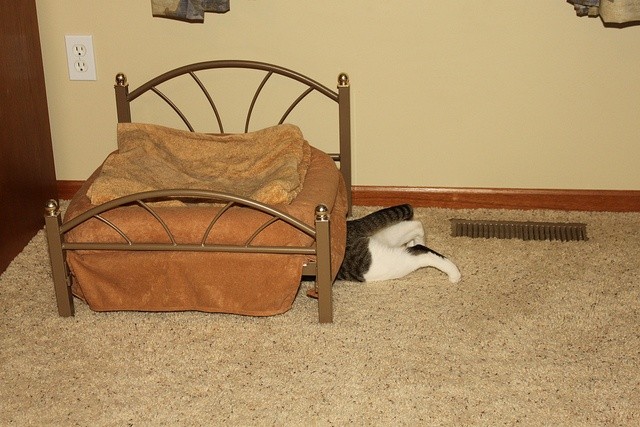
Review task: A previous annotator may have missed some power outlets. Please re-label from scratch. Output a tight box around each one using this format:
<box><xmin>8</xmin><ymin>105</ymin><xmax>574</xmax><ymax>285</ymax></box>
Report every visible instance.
<box><xmin>64</xmin><ymin>34</ymin><xmax>97</xmax><ymax>81</ymax></box>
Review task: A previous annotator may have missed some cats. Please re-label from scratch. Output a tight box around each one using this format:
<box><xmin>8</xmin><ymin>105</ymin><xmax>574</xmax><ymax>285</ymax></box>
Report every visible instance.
<box><xmin>337</xmin><ymin>203</ymin><xmax>462</xmax><ymax>284</ymax></box>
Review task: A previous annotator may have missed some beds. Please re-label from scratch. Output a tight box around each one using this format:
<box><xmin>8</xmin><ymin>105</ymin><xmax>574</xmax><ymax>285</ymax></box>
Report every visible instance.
<box><xmin>43</xmin><ymin>58</ymin><xmax>352</xmax><ymax>326</ymax></box>
